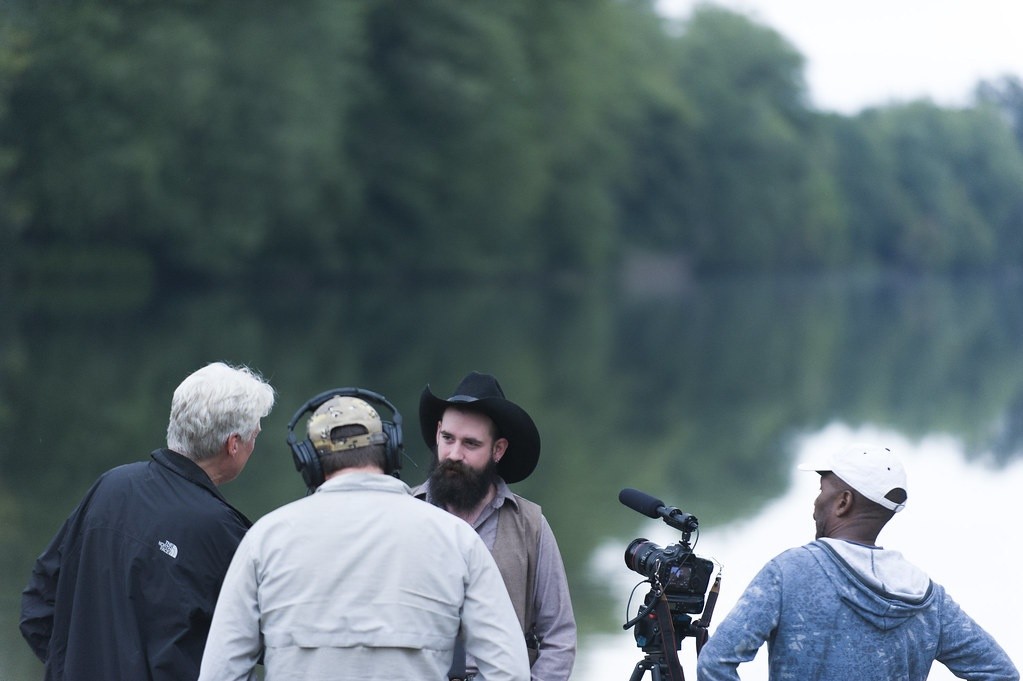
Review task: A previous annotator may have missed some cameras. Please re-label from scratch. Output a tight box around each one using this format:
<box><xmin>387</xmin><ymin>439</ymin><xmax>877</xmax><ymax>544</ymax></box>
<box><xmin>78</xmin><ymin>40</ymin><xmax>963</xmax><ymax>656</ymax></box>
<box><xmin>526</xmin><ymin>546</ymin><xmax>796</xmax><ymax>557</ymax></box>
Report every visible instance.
<box><xmin>625</xmin><ymin>538</ymin><xmax>713</xmax><ymax>614</ymax></box>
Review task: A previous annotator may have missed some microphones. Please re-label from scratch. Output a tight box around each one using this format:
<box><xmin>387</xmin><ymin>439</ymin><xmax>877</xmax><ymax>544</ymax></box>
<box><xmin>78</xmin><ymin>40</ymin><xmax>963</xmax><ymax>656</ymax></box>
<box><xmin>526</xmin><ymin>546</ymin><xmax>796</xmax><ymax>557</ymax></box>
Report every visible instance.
<box><xmin>619</xmin><ymin>488</ymin><xmax>699</xmax><ymax>528</ymax></box>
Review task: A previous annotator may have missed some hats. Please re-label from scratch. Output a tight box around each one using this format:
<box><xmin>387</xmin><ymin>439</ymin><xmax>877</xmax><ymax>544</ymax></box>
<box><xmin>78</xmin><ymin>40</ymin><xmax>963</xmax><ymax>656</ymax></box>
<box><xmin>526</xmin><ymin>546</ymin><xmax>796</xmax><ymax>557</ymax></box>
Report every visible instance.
<box><xmin>798</xmin><ymin>444</ymin><xmax>908</xmax><ymax>512</ymax></box>
<box><xmin>306</xmin><ymin>394</ymin><xmax>388</xmax><ymax>455</ymax></box>
<box><xmin>419</xmin><ymin>371</ymin><xmax>540</xmax><ymax>484</ymax></box>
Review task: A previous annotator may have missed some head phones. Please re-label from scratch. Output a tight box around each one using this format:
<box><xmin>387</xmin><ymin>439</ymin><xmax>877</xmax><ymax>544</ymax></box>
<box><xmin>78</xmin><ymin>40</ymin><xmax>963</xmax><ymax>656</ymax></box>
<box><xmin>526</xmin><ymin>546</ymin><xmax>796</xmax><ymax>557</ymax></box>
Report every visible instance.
<box><xmin>288</xmin><ymin>387</ymin><xmax>404</xmax><ymax>494</ymax></box>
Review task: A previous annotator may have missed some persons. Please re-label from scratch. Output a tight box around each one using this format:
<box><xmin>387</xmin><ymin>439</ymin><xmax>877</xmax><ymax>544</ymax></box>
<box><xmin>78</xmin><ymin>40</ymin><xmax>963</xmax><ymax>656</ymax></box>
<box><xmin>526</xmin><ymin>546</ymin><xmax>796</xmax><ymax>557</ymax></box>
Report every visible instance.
<box><xmin>195</xmin><ymin>396</ymin><xmax>531</xmax><ymax>681</ymax></box>
<box><xmin>406</xmin><ymin>370</ymin><xmax>577</xmax><ymax>680</ymax></box>
<box><xmin>19</xmin><ymin>360</ymin><xmax>278</xmax><ymax>681</ymax></box>
<box><xmin>697</xmin><ymin>444</ymin><xmax>1021</xmax><ymax>681</ymax></box>
